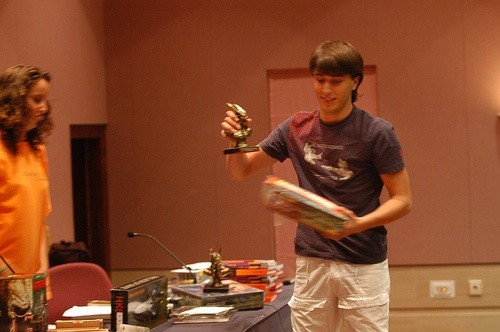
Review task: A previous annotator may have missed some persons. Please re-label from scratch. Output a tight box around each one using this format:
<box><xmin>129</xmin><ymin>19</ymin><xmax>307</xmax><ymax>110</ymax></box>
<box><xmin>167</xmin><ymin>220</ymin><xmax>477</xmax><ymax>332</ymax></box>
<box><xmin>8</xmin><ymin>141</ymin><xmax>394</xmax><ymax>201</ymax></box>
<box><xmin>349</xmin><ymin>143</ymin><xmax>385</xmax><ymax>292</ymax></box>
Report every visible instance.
<box><xmin>218</xmin><ymin>38</ymin><xmax>413</xmax><ymax>330</ymax></box>
<box><xmin>0</xmin><ymin>63</ymin><xmax>53</xmax><ymax>300</ymax></box>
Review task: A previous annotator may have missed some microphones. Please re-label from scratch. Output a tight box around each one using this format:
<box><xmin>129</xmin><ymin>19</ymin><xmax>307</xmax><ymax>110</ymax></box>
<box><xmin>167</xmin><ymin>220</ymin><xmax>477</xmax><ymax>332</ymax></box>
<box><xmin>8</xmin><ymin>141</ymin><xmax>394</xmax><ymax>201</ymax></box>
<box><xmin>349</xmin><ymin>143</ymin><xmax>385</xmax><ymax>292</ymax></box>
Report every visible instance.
<box><xmin>127</xmin><ymin>231</ymin><xmax>197</xmax><ymax>283</ymax></box>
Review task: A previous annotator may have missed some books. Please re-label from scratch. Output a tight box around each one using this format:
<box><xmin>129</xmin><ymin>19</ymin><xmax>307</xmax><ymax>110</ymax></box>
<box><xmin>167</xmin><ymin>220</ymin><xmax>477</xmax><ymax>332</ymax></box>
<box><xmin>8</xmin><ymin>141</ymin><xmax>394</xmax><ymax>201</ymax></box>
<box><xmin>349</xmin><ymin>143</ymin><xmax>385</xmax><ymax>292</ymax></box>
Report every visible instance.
<box><xmin>176</xmin><ymin>258</ymin><xmax>284</xmax><ymax>304</ymax></box>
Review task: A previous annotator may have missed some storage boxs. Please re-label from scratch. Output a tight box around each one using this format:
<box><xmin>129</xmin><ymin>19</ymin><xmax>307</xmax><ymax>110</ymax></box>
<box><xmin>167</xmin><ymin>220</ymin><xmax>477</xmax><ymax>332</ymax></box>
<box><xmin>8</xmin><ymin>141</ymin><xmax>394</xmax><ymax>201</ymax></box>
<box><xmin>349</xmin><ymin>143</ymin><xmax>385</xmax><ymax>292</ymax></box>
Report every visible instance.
<box><xmin>260</xmin><ymin>176</ymin><xmax>347</xmax><ymax>232</ymax></box>
<box><xmin>0</xmin><ymin>272</ymin><xmax>49</xmax><ymax>332</ymax></box>
<box><xmin>172</xmin><ymin>279</ymin><xmax>264</xmax><ymax>310</ymax></box>
<box><xmin>111</xmin><ymin>275</ymin><xmax>168</xmax><ymax>332</ymax></box>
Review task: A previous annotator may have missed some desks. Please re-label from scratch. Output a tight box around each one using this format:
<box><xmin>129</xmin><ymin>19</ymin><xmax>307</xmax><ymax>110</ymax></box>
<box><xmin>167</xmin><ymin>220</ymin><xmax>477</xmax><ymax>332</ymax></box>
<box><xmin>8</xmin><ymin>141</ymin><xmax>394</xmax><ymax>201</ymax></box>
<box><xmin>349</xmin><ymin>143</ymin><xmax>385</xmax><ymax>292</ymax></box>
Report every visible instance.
<box><xmin>151</xmin><ymin>283</ymin><xmax>293</xmax><ymax>332</ymax></box>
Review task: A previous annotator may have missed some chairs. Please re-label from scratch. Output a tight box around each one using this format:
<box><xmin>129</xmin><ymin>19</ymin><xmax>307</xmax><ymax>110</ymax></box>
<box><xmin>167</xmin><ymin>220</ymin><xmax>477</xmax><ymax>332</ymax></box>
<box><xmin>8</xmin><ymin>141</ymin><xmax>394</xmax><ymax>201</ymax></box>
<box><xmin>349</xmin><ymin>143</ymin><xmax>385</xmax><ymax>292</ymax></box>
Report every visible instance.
<box><xmin>44</xmin><ymin>263</ymin><xmax>112</xmax><ymax>325</ymax></box>
